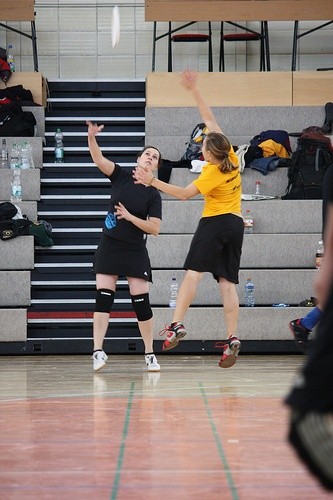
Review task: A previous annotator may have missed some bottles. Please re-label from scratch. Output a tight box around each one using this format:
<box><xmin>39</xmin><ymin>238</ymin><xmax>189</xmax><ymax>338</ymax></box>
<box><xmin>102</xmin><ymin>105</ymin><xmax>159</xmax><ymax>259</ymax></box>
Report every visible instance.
<box><xmin>55</xmin><ymin>128</ymin><xmax>64</xmax><ymax>163</ymax></box>
<box><xmin>11</xmin><ymin>165</ymin><xmax>23</xmax><ymax>202</ymax></box>
<box><xmin>6</xmin><ymin>45</ymin><xmax>15</xmax><ymax>72</ymax></box>
<box><xmin>254</xmin><ymin>181</ymin><xmax>261</xmax><ymax>201</ymax></box>
<box><xmin>245</xmin><ymin>277</ymin><xmax>255</xmax><ymax>307</ymax></box>
<box><xmin>244</xmin><ymin>210</ymin><xmax>254</xmax><ymax>234</ymax></box>
<box><xmin>20</xmin><ymin>142</ymin><xmax>30</xmax><ymax>168</ymax></box>
<box><xmin>10</xmin><ymin>143</ymin><xmax>19</xmax><ymax>169</ymax></box>
<box><xmin>168</xmin><ymin>277</ymin><xmax>179</xmax><ymax>308</ymax></box>
<box><xmin>316</xmin><ymin>241</ymin><xmax>324</xmax><ymax>269</ymax></box>
<box><xmin>0</xmin><ymin>139</ymin><xmax>10</xmax><ymax>169</ymax></box>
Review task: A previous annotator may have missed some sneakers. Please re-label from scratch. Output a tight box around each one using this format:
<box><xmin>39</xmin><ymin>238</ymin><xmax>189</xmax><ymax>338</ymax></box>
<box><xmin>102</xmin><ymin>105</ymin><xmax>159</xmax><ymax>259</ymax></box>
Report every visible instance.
<box><xmin>289</xmin><ymin>318</ymin><xmax>313</xmax><ymax>352</ymax></box>
<box><xmin>145</xmin><ymin>353</ymin><xmax>160</xmax><ymax>371</ymax></box>
<box><xmin>159</xmin><ymin>321</ymin><xmax>186</xmax><ymax>351</ymax></box>
<box><xmin>214</xmin><ymin>334</ymin><xmax>241</xmax><ymax>368</ymax></box>
<box><xmin>92</xmin><ymin>349</ymin><xmax>108</xmax><ymax>370</ymax></box>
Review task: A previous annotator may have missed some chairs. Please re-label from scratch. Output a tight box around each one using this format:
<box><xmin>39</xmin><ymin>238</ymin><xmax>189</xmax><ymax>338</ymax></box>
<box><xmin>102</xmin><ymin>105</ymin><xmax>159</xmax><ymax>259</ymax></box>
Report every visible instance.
<box><xmin>168</xmin><ymin>21</ymin><xmax>213</xmax><ymax>72</ymax></box>
<box><xmin>219</xmin><ymin>20</ymin><xmax>271</xmax><ymax>72</ymax></box>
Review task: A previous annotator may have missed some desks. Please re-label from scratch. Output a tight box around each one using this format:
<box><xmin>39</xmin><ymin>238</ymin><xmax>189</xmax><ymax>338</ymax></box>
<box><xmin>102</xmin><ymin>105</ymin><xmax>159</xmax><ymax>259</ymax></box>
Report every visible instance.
<box><xmin>0</xmin><ymin>0</ymin><xmax>38</xmax><ymax>73</ymax></box>
<box><xmin>144</xmin><ymin>0</ymin><xmax>333</xmax><ymax>74</ymax></box>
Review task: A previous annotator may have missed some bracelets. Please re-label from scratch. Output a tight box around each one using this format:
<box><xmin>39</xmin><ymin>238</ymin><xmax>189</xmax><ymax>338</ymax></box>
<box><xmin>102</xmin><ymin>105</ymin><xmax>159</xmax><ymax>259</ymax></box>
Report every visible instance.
<box><xmin>150</xmin><ymin>176</ymin><xmax>155</xmax><ymax>184</ymax></box>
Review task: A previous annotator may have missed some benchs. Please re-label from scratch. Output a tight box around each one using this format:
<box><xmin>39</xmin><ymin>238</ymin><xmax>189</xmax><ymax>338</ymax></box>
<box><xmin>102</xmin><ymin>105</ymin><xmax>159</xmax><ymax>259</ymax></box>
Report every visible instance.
<box><xmin>144</xmin><ymin>70</ymin><xmax>333</xmax><ymax>341</ymax></box>
<box><xmin>0</xmin><ymin>71</ymin><xmax>47</xmax><ymax>342</ymax></box>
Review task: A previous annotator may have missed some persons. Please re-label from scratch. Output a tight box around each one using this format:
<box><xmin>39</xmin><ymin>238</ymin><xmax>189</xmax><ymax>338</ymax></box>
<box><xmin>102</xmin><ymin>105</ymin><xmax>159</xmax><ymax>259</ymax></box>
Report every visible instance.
<box><xmin>131</xmin><ymin>71</ymin><xmax>245</xmax><ymax>368</ymax></box>
<box><xmin>290</xmin><ymin>160</ymin><xmax>333</xmax><ymax>351</ymax></box>
<box><xmin>85</xmin><ymin>122</ymin><xmax>162</xmax><ymax>372</ymax></box>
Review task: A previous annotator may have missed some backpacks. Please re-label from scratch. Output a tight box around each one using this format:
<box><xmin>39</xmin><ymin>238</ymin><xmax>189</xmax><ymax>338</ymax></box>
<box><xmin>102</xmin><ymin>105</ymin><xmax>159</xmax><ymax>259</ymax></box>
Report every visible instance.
<box><xmin>281</xmin><ymin>132</ymin><xmax>333</xmax><ymax>200</ymax></box>
